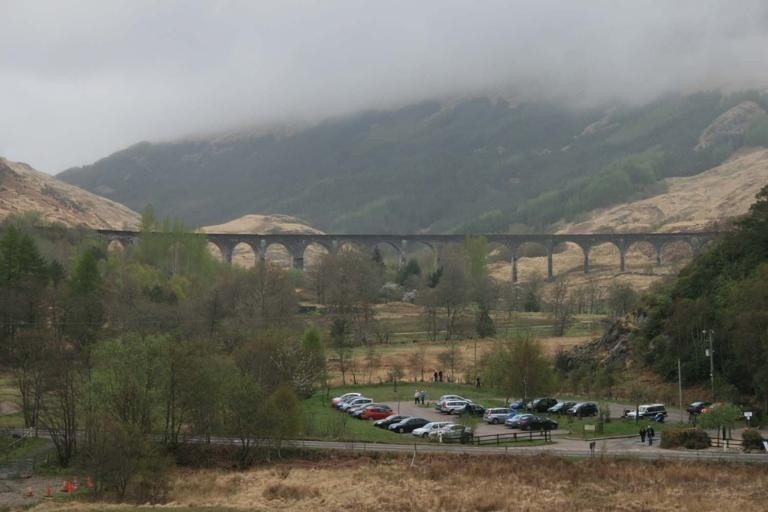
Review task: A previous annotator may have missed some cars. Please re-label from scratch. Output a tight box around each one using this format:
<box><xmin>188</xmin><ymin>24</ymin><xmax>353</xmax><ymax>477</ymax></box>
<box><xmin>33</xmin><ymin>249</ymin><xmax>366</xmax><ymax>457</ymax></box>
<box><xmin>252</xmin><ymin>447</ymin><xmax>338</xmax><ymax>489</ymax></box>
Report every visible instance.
<box><xmin>332</xmin><ymin>392</ymin><xmax>396</xmax><ymax>420</ymax></box>
<box><xmin>388</xmin><ymin>417</ymin><xmax>430</xmax><ymax>434</ymax></box>
<box><xmin>412</xmin><ymin>422</ymin><xmax>454</xmax><ymax>439</ymax></box>
<box><xmin>525</xmin><ymin>398</ymin><xmax>558</xmax><ymax>414</ymax></box>
<box><xmin>686</xmin><ymin>401</ymin><xmax>712</xmax><ymax>415</ymax></box>
<box><xmin>374</xmin><ymin>415</ymin><xmax>409</xmax><ymax>430</ymax></box>
<box><xmin>508</xmin><ymin>397</ymin><xmax>534</xmax><ymax>410</ymax></box>
<box><xmin>505</xmin><ymin>411</ymin><xmax>536</xmax><ymax>428</ymax></box>
<box><xmin>455</xmin><ymin>403</ymin><xmax>486</xmax><ymax>415</ymax></box>
<box><xmin>428</xmin><ymin>424</ymin><xmax>474</xmax><ymax>440</ymax></box>
<box><xmin>519</xmin><ymin>415</ymin><xmax>559</xmax><ymax>432</ymax></box>
<box><xmin>547</xmin><ymin>400</ymin><xmax>577</xmax><ymax>414</ymax></box>
<box><xmin>700</xmin><ymin>401</ymin><xmax>728</xmax><ymax>416</ymax></box>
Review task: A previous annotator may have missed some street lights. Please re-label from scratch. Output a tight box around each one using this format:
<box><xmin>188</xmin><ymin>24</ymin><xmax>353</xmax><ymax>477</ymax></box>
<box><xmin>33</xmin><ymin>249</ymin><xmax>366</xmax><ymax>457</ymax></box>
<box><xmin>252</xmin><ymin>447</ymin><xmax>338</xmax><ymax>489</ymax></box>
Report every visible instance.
<box><xmin>701</xmin><ymin>327</ymin><xmax>715</xmax><ymax>400</ymax></box>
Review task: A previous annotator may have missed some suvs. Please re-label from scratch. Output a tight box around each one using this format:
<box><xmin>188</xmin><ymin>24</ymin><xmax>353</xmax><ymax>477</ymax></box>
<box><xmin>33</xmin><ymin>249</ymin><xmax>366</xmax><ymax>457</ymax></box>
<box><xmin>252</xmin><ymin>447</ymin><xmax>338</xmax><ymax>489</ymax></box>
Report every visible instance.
<box><xmin>483</xmin><ymin>407</ymin><xmax>518</xmax><ymax>425</ymax></box>
<box><xmin>568</xmin><ymin>402</ymin><xmax>598</xmax><ymax>417</ymax></box>
<box><xmin>440</xmin><ymin>399</ymin><xmax>472</xmax><ymax>415</ymax></box>
<box><xmin>627</xmin><ymin>404</ymin><xmax>668</xmax><ymax>421</ymax></box>
<box><xmin>438</xmin><ymin>395</ymin><xmax>472</xmax><ymax>406</ymax></box>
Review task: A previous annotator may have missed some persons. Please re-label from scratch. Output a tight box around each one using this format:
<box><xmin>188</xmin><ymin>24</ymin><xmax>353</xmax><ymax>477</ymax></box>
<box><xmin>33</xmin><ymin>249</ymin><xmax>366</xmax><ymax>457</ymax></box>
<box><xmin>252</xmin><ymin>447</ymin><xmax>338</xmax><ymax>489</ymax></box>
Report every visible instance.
<box><xmin>433</xmin><ymin>369</ymin><xmax>438</xmax><ymax>382</ymax></box>
<box><xmin>420</xmin><ymin>390</ymin><xmax>424</xmax><ymax>405</ymax></box>
<box><xmin>476</xmin><ymin>375</ymin><xmax>481</xmax><ymax>389</ymax></box>
<box><xmin>414</xmin><ymin>390</ymin><xmax>420</xmax><ymax>405</ymax></box>
<box><xmin>640</xmin><ymin>425</ymin><xmax>646</xmax><ymax>441</ymax></box>
<box><xmin>438</xmin><ymin>369</ymin><xmax>443</xmax><ymax>381</ymax></box>
<box><xmin>646</xmin><ymin>425</ymin><xmax>655</xmax><ymax>445</ymax></box>
